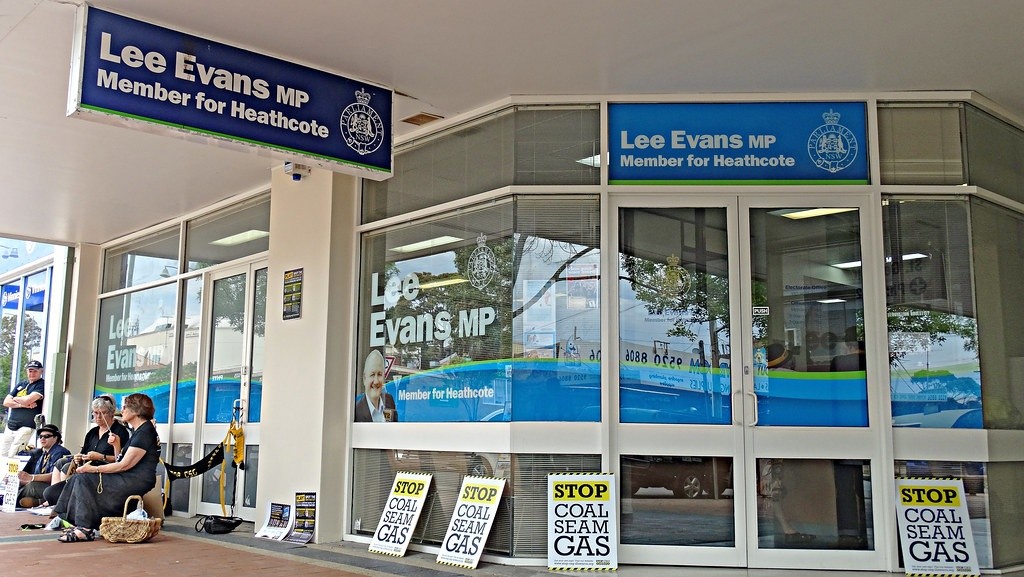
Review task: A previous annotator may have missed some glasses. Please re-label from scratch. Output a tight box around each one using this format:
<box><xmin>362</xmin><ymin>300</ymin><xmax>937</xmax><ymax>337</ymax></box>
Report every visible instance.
<box><xmin>40</xmin><ymin>434</ymin><xmax>54</xmax><ymax>438</ymax></box>
<box><xmin>94</xmin><ymin>396</ymin><xmax>113</xmax><ymax>405</ymax></box>
<box><xmin>123</xmin><ymin>406</ymin><xmax>130</xmax><ymax>409</ymax></box>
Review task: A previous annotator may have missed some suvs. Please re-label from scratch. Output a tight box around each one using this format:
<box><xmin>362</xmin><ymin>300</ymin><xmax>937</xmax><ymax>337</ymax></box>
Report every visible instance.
<box><xmin>577</xmin><ymin>406</ymin><xmax>733</xmax><ymax>498</ymax></box>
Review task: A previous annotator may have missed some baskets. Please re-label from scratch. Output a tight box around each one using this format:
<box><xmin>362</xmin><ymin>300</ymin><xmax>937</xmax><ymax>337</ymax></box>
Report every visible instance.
<box><xmin>99</xmin><ymin>495</ymin><xmax>161</xmax><ymax>543</ymax></box>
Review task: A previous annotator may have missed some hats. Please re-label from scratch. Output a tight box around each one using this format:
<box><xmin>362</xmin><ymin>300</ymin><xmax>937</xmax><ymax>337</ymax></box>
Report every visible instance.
<box><xmin>37</xmin><ymin>424</ymin><xmax>61</xmax><ymax>439</ymax></box>
<box><xmin>28</xmin><ymin>361</ymin><xmax>43</xmax><ymax>369</ymax></box>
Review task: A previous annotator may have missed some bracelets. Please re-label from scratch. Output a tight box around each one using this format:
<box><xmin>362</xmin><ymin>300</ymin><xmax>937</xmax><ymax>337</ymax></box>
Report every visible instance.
<box><xmin>31</xmin><ymin>475</ymin><xmax>35</xmax><ymax>481</ymax></box>
<box><xmin>19</xmin><ymin>404</ymin><xmax>21</xmax><ymax>408</ymax></box>
<box><xmin>103</xmin><ymin>454</ymin><xmax>107</xmax><ymax>461</ymax></box>
<box><xmin>96</xmin><ymin>466</ymin><xmax>100</xmax><ymax>473</ymax></box>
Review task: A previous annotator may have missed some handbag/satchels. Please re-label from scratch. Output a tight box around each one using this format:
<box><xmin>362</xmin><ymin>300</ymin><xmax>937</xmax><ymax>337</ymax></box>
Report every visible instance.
<box><xmin>193</xmin><ymin>514</ymin><xmax>243</xmax><ymax>536</ymax></box>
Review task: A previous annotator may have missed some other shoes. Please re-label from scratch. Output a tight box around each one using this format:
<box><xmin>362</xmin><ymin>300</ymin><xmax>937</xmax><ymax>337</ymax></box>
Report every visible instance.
<box><xmin>20</xmin><ymin>497</ymin><xmax>45</xmax><ymax>508</ymax></box>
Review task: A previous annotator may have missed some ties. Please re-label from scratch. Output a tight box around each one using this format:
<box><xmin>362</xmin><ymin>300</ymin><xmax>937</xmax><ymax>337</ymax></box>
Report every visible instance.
<box><xmin>34</xmin><ymin>450</ymin><xmax>46</xmax><ymax>474</ymax></box>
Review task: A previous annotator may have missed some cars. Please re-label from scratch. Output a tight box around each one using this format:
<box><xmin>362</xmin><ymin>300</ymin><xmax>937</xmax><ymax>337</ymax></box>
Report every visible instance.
<box><xmin>457</xmin><ymin>408</ymin><xmax>512</xmax><ymax>485</ymax></box>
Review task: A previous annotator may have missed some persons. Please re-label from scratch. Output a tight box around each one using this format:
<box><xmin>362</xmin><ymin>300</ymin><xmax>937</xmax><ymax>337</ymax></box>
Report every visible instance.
<box><xmin>827</xmin><ymin>326</ymin><xmax>866</xmax><ymax>547</ymax></box>
<box><xmin>38</xmin><ymin>393</ymin><xmax>160</xmax><ymax>542</ymax></box>
<box><xmin>754</xmin><ymin>343</ymin><xmax>814</xmax><ymax>544</ymax></box>
<box><xmin>0</xmin><ymin>360</ymin><xmax>44</xmax><ymax>456</ymax></box>
<box><xmin>3</xmin><ymin>424</ymin><xmax>72</xmax><ymax>507</ymax></box>
<box><xmin>354</xmin><ymin>350</ymin><xmax>397</xmax><ymax>422</ymax></box>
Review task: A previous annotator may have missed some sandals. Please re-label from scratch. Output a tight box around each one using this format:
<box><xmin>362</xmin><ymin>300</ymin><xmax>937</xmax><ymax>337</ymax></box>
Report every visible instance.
<box><xmin>58</xmin><ymin>527</ymin><xmax>95</xmax><ymax>542</ymax></box>
